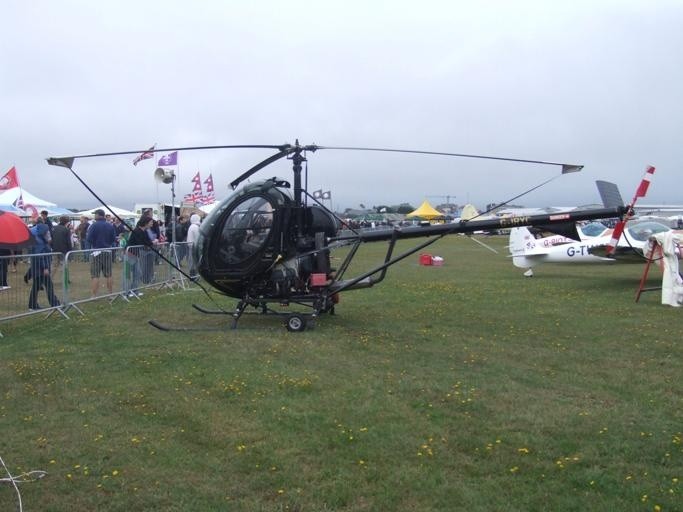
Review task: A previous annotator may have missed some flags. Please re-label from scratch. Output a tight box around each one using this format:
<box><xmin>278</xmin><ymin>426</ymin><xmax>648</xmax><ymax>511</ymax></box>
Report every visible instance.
<box><xmin>132</xmin><ymin>148</ymin><xmax>153</xmax><ymax>167</ymax></box>
<box><xmin>0</xmin><ymin>166</ymin><xmax>19</xmax><ymax>191</ymax></box>
<box><xmin>11</xmin><ymin>194</ymin><xmax>27</xmax><ymax>215</ymax></box>
<box><xmin>156</xmin><ymin>151</ymin><xmax>177</xmax><ymax>166</ymax></box>
<box><xmin>312</xmin><ymin>189</ymin><xmax>321</xmax><ymax>200</ymax></box>
<box><xmin>183</xmin><ymin>192</ymin><xmax>204</xmax><ymax>209</ymax></box>
<box><xmin>319</xmin><ymin>191</ymin><xmax>330</xmax><ymax>200</ymax></box>
<box><xmin>197</xmin><ymin>194</ymin><xmax>215</xmax><ymax>206</ymax></box>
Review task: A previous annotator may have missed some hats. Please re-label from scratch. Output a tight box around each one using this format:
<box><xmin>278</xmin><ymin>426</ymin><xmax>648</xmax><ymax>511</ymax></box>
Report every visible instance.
<box><xmin>91</xmin><ymin>210</ymin><xmax>104</xmax><ymax>214</ymax></box>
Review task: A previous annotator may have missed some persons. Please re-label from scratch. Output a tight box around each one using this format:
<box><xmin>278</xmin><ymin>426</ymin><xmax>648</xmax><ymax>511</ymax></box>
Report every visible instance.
<box><xmin>336</xmin><ymin>218</ymin><xmax>387</xmax><ymax>243</ymax></box>
<box><xmin>0</xmin><ymin>208</ymin><xmax>207</xmax><ymax>310</ymax></box>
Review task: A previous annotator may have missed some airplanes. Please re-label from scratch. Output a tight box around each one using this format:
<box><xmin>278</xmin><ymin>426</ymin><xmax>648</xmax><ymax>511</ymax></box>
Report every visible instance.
<box><xmin>481</xmin><ymin>200</ymin><xmax>683</xmax><ymax>279</ymax></box>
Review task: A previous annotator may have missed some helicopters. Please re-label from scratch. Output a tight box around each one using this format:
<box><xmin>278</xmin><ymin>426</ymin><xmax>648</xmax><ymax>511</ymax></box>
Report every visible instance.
<box><xmin>37</xmin><ymin>138</ymin><xmax>638</xmax><ymax>338</ymax></box>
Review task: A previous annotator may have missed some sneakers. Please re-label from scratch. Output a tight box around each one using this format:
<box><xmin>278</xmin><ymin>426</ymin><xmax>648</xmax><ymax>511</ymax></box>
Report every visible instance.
<box><xmin>128</xmin><ymin>293</ymin><xmax>134</xmax><ymax>297</ymax></box>
<box><xmin>138</xmin><ymin>293</ymin><xmax>143</xmax><ymax>295</ymax></box>
<box><xmin>29</xmin><ymin>306</ymin><xmax>41</xmax><ymax>311</ymax></box>
<box><xmin>24</xmin><ymin>275</ymin><xmax>28</xmax><ymax>283</ymax></box>
